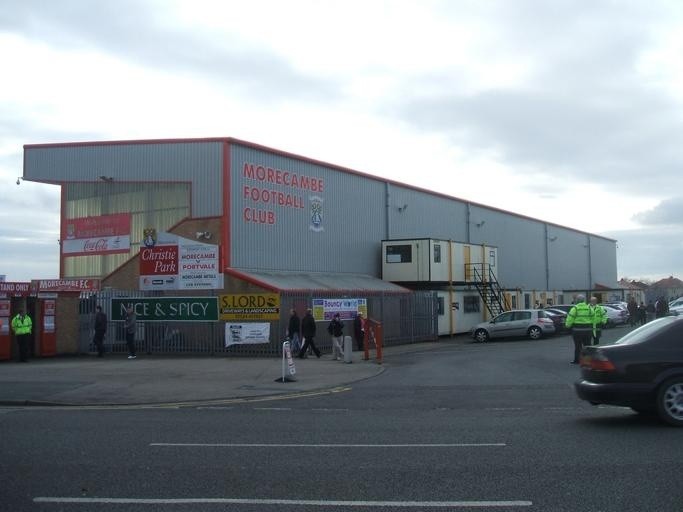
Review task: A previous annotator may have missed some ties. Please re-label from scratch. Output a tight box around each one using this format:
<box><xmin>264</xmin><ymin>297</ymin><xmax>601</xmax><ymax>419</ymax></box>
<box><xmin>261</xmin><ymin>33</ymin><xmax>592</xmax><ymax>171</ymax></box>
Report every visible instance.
<box><xmin>21</xmin><ymin>316</ymin><xmax>24</xmax><ymax>324</ymax></box>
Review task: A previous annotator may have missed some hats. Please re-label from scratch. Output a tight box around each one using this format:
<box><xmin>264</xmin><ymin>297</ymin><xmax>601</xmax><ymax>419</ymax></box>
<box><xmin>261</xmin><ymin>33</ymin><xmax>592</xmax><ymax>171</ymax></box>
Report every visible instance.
<box><xmin>18</xmin><ymin>308</ymin><xmax>25</xmax><ymax>312</ymax></box>
<box><xmin>357</xmin><ymin>311</ymin><xmax>363</xmax><ymax>315</ymax></box>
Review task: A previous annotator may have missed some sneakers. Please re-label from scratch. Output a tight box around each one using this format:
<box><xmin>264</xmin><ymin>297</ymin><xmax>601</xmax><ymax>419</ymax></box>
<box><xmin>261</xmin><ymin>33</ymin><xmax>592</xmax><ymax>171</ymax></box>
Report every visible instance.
<box><xmin>284</xmin><ymin>353</ymin><xmax>344</xmax><ymax>361</ymax></box>
<box><xmin>95</xmin><ymin>354</ymin><xmax>137</xmax><ymax>359</ymax></box>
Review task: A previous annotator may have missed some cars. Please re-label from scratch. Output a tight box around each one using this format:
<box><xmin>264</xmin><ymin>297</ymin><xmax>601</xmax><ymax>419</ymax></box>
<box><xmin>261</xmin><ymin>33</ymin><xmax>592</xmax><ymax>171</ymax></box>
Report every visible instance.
<box><xmin>469</xmin><ymin>295</ymin><xmax>683</xmax><ymax>342</ymax></box>
<box><xmin>574</xmin><ymin>313</ymin><xmax>682</xmax><ymax>430</ymax></box>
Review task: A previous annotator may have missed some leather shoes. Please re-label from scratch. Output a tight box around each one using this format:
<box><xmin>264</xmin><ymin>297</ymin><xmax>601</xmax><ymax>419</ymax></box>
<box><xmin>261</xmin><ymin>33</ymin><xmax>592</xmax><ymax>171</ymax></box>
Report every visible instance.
<box><xmin>570</xmin><ymin>359</ymin><xmax>579</xmax><ymax>364</ymax></box>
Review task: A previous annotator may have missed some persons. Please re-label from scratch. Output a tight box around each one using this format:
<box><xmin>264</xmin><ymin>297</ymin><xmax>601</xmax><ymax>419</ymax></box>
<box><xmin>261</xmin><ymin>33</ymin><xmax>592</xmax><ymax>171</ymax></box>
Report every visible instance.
<box><xmin>654</xmin><ymin>294</ymin><xmax>682</xmax><ymax>320</ymax></box>
<box><xmin>637</xmin><ymin>300</ymin><xmax>647</xmax><ymax>326</ymax></box>
<box><xmin>625</xmin><ymin>296</ymin><xmax>638</xmax><ymax>327</ymax></box>
<box><xmin>93</xmin><ymin>305</ymin><xmax>107</xmax><ymax>358</ymax></box>
<box><xmin>645</xmin><ymin>299</ymin><xmax>655</xmax><ymax>323</ymax></box>
<box><xmin>564</xmin><ymin>294</ymin><xmax>596</xmax><ymax>364</ymax></box>
<box><xmin>294</xmin><ymin>307</ymin><xmax>321</xmax><ymax>359</ymax></box>
<box><xmin>587</xmin><ymin>296</ymin><xmax>608</xmax><ymax>346</ymax></box>
<box><xmin>532</xmin><ymin>300</ymin><xmax>542</xmax><ymax>309</ymax></box>
<box><xmin>287</xmin><ymin>307</ymin><xmax>300</xmax><ymax>353</ymax></box>
<box><xmin>353</xmin><ymin>311</ymin><xmax>368</xmax><ymax>351</ymax></box>
<box><xmin>326</xmin><ymin>313</ymin><xmax>344</xmax><ymax>361</ymax></box>
<box><xmin>122</xmin><ymin>306</ymin><xmax>136</xmax><ymax>360</ymax></box>
<box><xmin>10</xmin><ymin>308</ymin><xmax>34</xmax><ymax>362</ymax></box>
